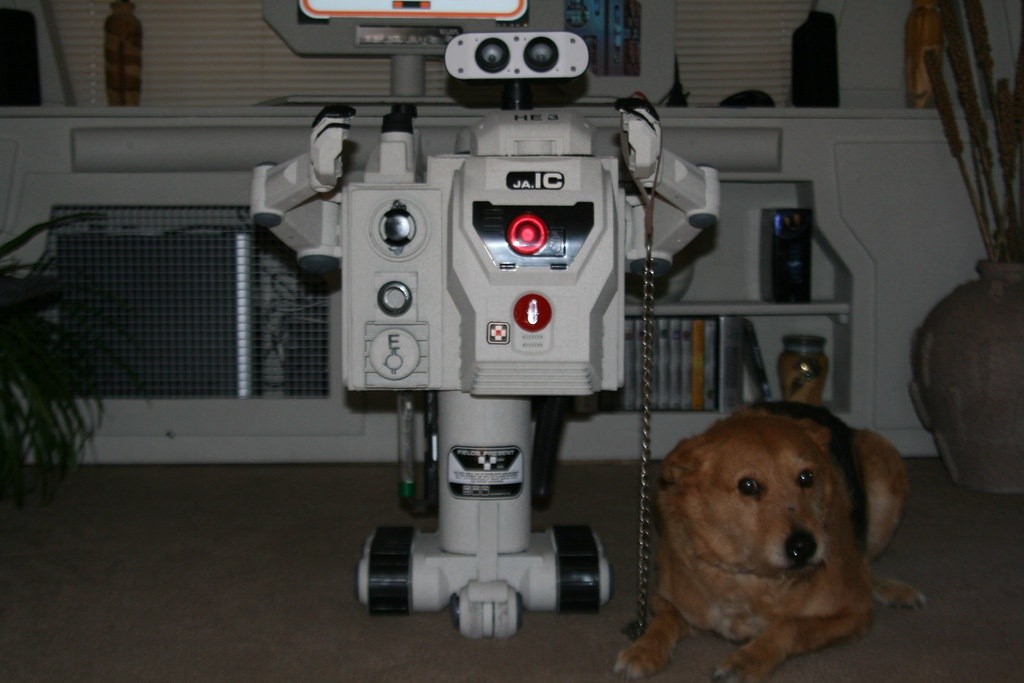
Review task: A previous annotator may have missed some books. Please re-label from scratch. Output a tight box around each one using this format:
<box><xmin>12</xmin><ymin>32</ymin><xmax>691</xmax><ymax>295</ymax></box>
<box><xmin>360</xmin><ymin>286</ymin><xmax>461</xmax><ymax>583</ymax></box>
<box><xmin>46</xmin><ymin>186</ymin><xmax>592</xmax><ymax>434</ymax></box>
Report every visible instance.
<box><xmin>598</xmin><ymin>315</ymin><xmax>771</xmax><ymax>412</ymax></box>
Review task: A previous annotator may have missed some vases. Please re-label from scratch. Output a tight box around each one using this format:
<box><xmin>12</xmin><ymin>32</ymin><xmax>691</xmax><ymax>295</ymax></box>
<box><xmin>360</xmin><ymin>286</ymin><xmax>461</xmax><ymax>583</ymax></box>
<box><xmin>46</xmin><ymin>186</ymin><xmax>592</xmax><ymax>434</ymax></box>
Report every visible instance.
<box><xmin>909</xmin><ymin>260</ymin><xmax>1024</xmax><ymax>494</ymax></box>
<box><xmin>105</xmin><ymin>2</ymin><xmax>143</xmax><ymax>105</ymax></box>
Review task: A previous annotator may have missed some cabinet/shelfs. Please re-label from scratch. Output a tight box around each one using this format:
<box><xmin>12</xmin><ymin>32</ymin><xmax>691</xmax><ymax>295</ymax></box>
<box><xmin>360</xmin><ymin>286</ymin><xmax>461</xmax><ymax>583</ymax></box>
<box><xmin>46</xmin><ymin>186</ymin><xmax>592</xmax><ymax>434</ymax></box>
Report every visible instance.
<box><xmin>0</xmin><ymin>106</ymin><xmax>1024</xmax><ymax>463</ymax></box>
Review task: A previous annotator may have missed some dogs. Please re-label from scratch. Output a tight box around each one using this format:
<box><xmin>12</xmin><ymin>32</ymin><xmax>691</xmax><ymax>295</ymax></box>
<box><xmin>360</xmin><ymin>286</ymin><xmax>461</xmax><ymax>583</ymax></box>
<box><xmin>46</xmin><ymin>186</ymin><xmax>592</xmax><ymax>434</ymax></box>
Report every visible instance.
<box><xmin>615</xmin><ymin>399</ymin><xmax>927</xmax><ymax>683</ymax></box>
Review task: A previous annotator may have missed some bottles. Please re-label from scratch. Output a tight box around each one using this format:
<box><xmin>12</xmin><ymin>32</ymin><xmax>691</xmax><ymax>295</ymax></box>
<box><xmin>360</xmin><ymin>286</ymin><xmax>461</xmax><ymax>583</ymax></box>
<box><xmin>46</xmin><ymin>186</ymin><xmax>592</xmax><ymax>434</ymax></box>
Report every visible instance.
<box><xmin>778</xmin><ymin>334</ymin><xmax>828</xmax><ymax>407</ymax></box>
<box><xmin>104</xmin><ymin>1</ymin><xmax>143</xmax><ymax>106</ymax></box>
